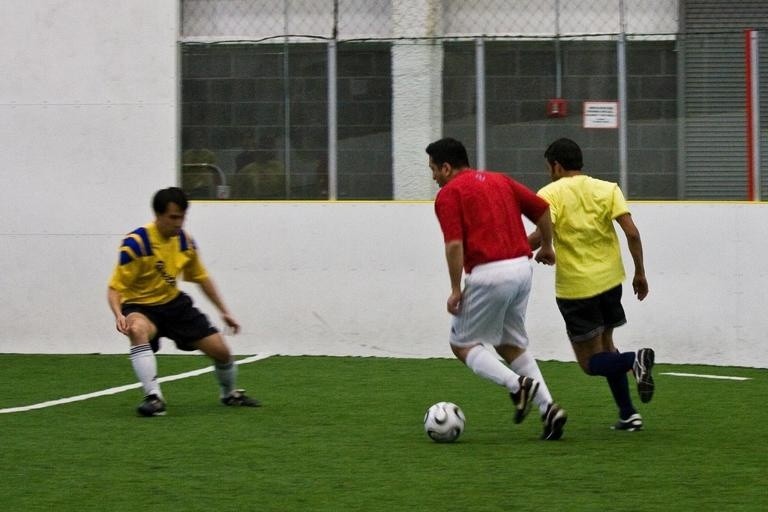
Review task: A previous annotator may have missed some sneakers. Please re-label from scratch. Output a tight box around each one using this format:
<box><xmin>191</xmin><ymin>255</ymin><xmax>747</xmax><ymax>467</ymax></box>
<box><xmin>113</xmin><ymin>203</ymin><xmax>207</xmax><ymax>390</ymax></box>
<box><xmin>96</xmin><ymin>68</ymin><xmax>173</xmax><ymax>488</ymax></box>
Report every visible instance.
<box><xmin>632</xmin><ymin>348</ymin><xmax>654</xmax><ymax>402</ymax></box>
<box><xmin>138</xmin><ymin>394</ymin><xmax>165</xmax><ymax>416</ymax></box>
<box><xmin>510</xmin><ymin>376</ymin><xmax>540</xmax><ymax>424</ymax></box>
<box><xmin>221</xmin><ymin>389</ymin><xmax>261</xmax><ymax>407</ymax></box>
<box><xmin>615</xmin><ymin>414</ymin><xmax>643</xmax><ymax>429</ymax></box>
<box><xmin>542</xmin><ymin>403</ymin><xmax>566</xmax><ymax>440</ymax></box>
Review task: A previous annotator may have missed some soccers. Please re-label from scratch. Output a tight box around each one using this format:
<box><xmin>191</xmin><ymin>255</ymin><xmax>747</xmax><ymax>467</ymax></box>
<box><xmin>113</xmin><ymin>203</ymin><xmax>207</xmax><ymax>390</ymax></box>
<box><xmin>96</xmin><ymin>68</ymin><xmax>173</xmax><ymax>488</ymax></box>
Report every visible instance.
<box><xmin>423</xmin><ymin>402</ymin><xmax>465</xmax><ymax>443</ymax></box>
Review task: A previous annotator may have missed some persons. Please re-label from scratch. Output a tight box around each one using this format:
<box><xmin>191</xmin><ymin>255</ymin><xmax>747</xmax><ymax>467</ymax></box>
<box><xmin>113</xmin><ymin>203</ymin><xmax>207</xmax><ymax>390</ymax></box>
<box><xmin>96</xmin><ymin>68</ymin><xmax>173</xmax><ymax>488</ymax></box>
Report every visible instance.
<box><xmin>424</xmin><ymin>138</ymin><xmax>565</xmax><ymax>440</ymax></box>
<box><xmin>106</xmin><ymin>186</ymin><xmax>263</xmax><ymax>418</ymax></box>
<box><xmin>182</xmin><ymin>128</ymin><xmax>330</xmax><ymax>199</ymax></box>
<box><xmin>526</xmin><ymin>137</ymin><xmax>654</xmax><ymax>433</ymax></box>
